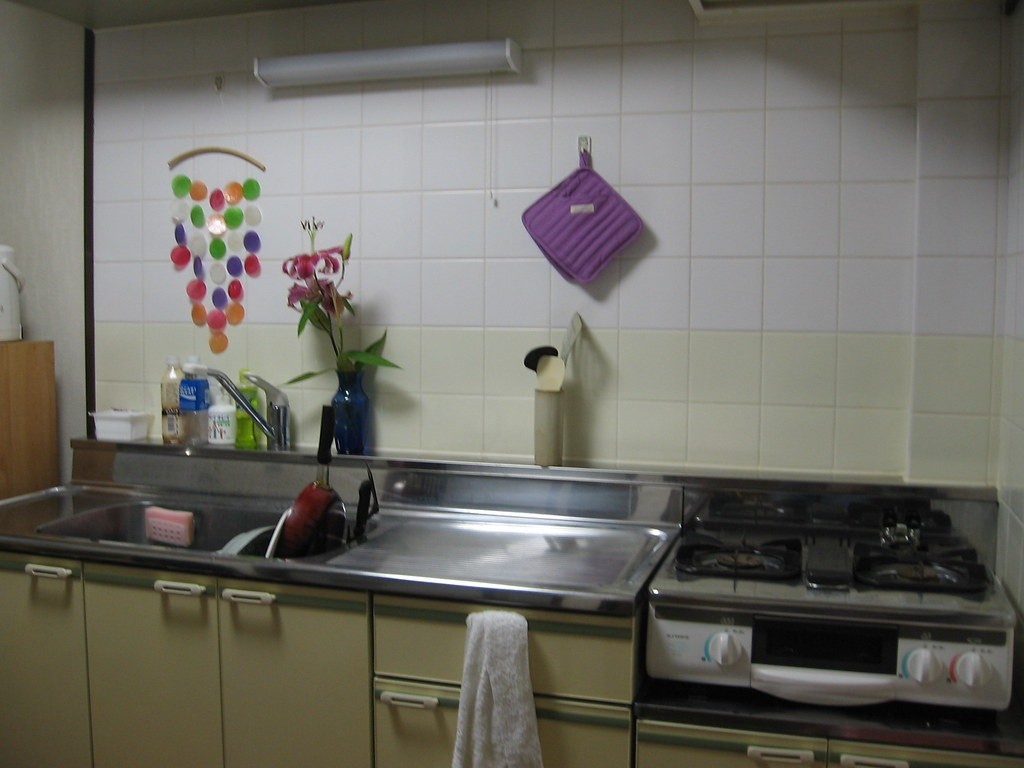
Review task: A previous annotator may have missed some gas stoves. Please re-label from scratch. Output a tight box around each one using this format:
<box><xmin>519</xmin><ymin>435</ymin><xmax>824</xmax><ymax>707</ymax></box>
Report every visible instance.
<box><xmin>646</xmin><ymin>485</ymin><xmax>1017</xmax><ymax>631</ymax></box>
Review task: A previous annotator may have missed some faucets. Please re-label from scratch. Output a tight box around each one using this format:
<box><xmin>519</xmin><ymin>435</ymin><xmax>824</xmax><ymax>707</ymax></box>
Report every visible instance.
<box><xmin>182</xmin><ymin>360</ymin><xmax>291</xmax><ymax>453</ymax></box>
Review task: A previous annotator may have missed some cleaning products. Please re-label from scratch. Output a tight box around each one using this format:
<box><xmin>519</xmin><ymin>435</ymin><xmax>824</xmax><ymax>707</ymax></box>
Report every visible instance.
<box><xmin>160</xmin><ymin>355</ymin><xmax>259</xmax><ymax>449</ymax></box>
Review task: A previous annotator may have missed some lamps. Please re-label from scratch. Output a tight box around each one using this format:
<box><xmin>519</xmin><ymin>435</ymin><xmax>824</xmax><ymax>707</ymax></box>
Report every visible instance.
<box><xmin>251</xmin><ymin>40</ymin><xmax>524</xmax><ymax>88</ymax></box>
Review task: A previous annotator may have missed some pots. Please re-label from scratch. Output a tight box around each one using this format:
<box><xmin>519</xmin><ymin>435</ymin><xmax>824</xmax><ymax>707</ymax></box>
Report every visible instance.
<box><xmin>0</xmin><ymin>245</ymin><xmax>25</xmax><ymax>342</ymax></box>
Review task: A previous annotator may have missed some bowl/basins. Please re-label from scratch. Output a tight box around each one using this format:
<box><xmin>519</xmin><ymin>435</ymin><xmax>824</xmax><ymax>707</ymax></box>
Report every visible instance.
<box><xmin>87</xmin><ymin>408</ymin><xmax>150</xmax><ymax>442</ymax></box>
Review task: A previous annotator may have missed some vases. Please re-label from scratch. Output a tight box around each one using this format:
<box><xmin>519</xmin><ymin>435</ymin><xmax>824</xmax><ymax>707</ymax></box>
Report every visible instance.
<box><xmin>332</xmin><ymin>369</ymin><xmax>371</xmax><ymax>454</ymax></box>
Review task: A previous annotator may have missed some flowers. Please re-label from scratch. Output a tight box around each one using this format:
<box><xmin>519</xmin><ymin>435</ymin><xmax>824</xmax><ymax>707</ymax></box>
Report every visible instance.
<box><xmin>281</xmin><ymin>217</ymin><xmax>403</xmax><ymax>391</ymax></box>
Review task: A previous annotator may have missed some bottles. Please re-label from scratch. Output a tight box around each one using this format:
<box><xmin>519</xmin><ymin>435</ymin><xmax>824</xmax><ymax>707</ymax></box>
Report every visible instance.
<box><xmin>178</xmin><ymin>354</ymin><xmax>210</xmax><ymax>446</ymax></box>
<box><xmin>159</xmin><ymin>355</ymin><xmax>185</xmax><ymax>444</ymax></box>
<box><xmin>234</xmin><ymin>369</ymin><xmax>262</xmax><ymax>449</ymax></box>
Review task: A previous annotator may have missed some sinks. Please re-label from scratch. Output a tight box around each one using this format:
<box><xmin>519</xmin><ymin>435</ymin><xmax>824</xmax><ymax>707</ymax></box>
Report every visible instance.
<box><xmin>0</xmin><ymin>487</ymin><xmax>152</xmax><ymax>555</ymax></box>
<box><xmin>34</xmin><ymin>498</ymin><xmax>383</xmax><ymax>567</ymax></box>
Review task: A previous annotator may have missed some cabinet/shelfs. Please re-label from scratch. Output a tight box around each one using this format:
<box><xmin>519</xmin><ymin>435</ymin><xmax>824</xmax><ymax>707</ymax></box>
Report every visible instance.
<box><xmin>1</xmin><ymin>549</ymin><xmax>1024</xmax><ymax>768</ymax></box>
